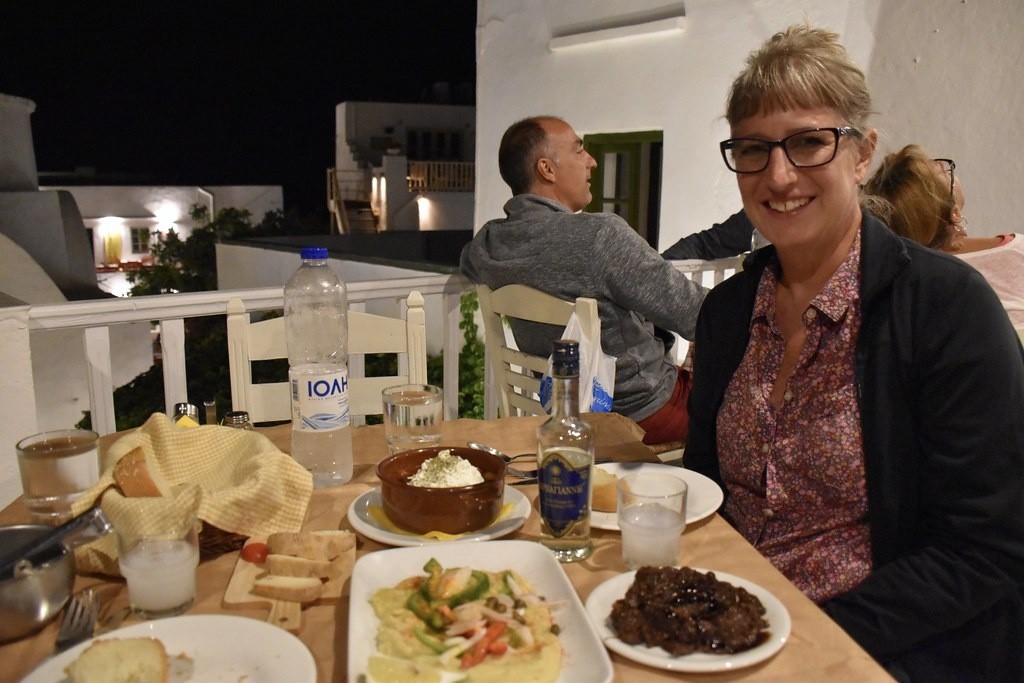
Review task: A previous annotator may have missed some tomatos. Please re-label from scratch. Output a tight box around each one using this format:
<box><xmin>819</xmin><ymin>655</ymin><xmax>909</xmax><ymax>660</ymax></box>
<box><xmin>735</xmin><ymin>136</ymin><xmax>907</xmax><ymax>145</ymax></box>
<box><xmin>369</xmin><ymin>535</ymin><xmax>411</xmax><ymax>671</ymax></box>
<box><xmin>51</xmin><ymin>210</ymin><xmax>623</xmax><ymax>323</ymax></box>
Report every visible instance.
<box><xmin>241</xmin><ymin>543</ymin><xmax>270</xmax><ymax>563</ymax></box>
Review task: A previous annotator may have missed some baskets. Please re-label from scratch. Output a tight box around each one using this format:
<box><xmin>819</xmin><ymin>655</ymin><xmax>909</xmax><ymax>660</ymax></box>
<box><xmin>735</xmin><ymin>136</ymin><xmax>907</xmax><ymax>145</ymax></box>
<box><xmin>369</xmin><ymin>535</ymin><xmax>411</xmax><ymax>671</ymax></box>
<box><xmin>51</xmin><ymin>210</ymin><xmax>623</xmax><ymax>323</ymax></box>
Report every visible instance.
<box><xmin>191</xmin><ymin>522</ymin><xmax>246</xmax><ymax>555</ymax></box>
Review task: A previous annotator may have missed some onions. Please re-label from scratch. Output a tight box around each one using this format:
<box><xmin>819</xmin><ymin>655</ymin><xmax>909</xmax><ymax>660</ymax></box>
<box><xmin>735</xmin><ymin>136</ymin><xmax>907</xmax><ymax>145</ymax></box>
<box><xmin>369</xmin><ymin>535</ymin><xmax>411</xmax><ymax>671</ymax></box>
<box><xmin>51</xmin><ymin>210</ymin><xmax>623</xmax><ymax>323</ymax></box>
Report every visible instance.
<box><xmin>439</xmin><ymin>574</ymin><xmax>534</xmax><ymax>668</ymax></box>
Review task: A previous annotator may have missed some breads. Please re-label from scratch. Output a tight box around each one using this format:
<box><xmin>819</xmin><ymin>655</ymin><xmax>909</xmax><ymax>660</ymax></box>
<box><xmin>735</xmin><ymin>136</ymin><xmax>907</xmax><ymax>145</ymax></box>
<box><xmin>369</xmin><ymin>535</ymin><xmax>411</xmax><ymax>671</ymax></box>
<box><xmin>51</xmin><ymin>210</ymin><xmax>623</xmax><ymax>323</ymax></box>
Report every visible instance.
<box><xmin>255</xmin><ymin>530</ymin><xmax>356</xmax><ymax>603</ymax></box>
<box><xmin>591</xmin><ymin>465</ymin><xmax>637</xmax><ymax>512</ymax></box>
<box><xmin>63</xmin><ymin>635</ymin><xmax>169</xmax><ymax>683</ymax></box>
<box><xmin>112</xmin><ymin>445</ymin><xmax>163</xmax><ymax>497</ymax></box>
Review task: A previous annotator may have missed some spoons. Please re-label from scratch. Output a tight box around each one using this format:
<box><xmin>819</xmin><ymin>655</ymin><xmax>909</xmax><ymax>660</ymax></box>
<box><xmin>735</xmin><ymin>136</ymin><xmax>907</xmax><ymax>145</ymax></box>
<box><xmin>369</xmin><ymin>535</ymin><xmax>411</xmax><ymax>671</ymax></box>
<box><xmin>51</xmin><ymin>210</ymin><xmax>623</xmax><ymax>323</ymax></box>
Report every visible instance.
<box><xmin>467</xmin><ymin>441</ymin><xmax>537</xmax><ymax>462</ymax></box>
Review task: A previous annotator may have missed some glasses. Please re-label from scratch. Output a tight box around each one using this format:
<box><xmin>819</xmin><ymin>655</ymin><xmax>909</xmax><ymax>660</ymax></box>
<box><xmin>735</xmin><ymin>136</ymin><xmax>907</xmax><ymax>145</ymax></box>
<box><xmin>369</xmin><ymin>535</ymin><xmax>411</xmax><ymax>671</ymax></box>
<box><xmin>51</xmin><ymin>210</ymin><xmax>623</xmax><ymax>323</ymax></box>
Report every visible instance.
<box><xmin>718</xmin><ymin>125</ymin><xmax>862</xmax><ymax>174</ymax></box>
<box><xmin>934</xmin><ymin>158</ymin><xmax>956</xmax><ymax>196</ymax></box>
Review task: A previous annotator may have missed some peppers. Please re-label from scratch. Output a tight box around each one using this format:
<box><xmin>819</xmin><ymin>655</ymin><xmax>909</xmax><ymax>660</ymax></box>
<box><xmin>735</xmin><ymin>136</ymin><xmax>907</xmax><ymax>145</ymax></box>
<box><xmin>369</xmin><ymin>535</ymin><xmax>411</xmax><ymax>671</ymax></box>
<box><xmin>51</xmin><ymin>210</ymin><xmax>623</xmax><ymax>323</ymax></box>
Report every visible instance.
<box><xmin>404</xmin><ymin>558</ymin><xmax>508</xmax><ymax>666</ymax></box>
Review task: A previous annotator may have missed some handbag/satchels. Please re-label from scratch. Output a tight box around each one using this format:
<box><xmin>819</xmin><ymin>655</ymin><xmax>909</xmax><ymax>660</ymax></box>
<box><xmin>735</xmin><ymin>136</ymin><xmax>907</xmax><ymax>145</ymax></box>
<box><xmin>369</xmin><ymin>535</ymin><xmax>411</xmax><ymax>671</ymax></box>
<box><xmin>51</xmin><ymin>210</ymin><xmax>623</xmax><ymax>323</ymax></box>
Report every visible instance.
<box><xmin>538</xmin><ymin>312</ymin><xmax>617</xmax><ymax>415</ymax></box>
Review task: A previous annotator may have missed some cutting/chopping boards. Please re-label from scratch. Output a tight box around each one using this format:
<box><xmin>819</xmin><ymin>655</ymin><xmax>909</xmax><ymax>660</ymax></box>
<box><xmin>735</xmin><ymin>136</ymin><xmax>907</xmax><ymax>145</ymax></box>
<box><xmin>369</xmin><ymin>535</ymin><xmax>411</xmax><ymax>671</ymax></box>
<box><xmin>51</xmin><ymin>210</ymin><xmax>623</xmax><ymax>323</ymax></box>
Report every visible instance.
<box><xmin>222</xmin><ymin>531</ymin><xmax>357</xmax><ymax>636</ymax></box>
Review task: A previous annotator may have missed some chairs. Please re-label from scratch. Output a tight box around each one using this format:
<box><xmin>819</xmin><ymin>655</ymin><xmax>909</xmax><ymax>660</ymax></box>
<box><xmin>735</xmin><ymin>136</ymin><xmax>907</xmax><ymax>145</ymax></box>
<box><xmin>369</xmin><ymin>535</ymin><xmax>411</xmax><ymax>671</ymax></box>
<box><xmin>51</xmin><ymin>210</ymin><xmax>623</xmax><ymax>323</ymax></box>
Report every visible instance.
<box><xmin>475</xmin><ymin>282</ymin><xmax>686</xmax><ymax>462</ymax></box>
<box><xmin>226</xmin><ymin>290</ymin><xmax>426</xmax><ymax>425</ymax></box>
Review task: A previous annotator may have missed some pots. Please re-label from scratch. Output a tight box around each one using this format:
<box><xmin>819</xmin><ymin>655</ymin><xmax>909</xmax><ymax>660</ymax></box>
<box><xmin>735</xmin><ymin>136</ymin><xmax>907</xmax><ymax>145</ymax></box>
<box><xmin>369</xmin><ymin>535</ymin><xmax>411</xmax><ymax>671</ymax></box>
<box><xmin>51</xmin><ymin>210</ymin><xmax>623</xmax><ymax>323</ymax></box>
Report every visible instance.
<box><xmin>1</xmin><ymin>523</ymin><xmax>75</xmax><ymax>644</ymax></box>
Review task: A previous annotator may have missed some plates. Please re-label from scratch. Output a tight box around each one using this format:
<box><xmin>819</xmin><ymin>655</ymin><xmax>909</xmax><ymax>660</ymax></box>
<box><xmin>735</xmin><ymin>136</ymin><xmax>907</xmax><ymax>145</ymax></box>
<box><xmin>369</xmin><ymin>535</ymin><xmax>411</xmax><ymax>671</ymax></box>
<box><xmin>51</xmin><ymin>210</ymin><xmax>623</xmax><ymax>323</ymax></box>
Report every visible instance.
<box><xmin>346</xmin><ymin>488</ymin><xmax>532</xmax><ymax>545</ymax></box>
<box><xmin>17</xmin><ymin>614</ymin><xmax>319</xmax><ymax>683</ymax></box>
<box><xmin>586</xmin><ymin>567</ymin><xmax>793</xmax><ymax>674</ymax></box>
<box><xmin>340</xmin><ymin>540</ymin><xmax>617</xmax><ymax>678</ymax></box>
<box><xmin>591</xmin><ymin>464</ymin><xmax>722</xmax><ymax>535</ymax></box>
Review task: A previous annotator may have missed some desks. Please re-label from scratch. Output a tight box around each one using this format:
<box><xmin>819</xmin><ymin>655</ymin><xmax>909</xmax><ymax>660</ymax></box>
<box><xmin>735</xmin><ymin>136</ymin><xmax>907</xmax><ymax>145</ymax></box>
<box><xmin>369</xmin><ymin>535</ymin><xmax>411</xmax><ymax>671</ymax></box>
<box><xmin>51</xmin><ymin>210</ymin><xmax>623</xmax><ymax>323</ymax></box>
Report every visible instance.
<box><xmin>0</xmin><ymin>411</ymin><xmax>897</xmax><ymax>683</ymax></box>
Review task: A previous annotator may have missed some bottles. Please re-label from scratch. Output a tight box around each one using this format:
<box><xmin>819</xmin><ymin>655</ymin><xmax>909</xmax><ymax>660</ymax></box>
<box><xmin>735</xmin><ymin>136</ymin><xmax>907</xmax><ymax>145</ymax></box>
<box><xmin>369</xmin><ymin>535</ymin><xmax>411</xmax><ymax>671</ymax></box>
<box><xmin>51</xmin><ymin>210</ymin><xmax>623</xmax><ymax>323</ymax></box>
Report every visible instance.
<box><xmin>283</xmin><ymin>243</ymin><xmax>354</xmax><ymax>486</ymax></box>
<box><xmin>225</xmin><ymin>412</ymin><xmax>255</xmax><ymax>432</ymax></box>
<box><xmin>171</xmin><ymin>403</ymin><xmax>201</xmax><ymax>429</ymax></box>
<box><xmin>533</xmin><ymin>339</ymin><xmax>597</xmax><ymax>563</ymax></box>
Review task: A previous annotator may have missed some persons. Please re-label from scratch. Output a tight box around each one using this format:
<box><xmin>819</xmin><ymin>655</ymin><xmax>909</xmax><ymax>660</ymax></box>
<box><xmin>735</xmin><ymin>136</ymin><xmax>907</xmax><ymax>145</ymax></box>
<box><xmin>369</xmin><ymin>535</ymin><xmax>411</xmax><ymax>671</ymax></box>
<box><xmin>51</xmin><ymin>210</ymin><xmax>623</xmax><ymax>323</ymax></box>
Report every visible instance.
<box><xmin>683</xmin><ymin>22</ymin><xmax>1024</xmax><ymax>683</ymax></box>
<box><xmin>458</xmin><ymin>115</ymin><xmax>713</xmax><ymax>447</ymax></box>
<box><xmin>655</xmin><ymin>203</ymin><xmax>772</xmax><ymax>262</ymax></box>
<box><xmin>863</xmin><ymin>143</ymin><xmax>1024</xmax><ymax>345</ymax></box>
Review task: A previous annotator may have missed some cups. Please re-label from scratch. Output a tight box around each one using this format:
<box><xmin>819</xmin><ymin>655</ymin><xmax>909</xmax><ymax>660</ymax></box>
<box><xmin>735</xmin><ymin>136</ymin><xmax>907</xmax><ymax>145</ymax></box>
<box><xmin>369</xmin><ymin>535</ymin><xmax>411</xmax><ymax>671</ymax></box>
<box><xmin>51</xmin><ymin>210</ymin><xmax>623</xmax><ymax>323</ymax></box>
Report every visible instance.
<box><xmin>616</xmin><ymin>469</ymin><xmax>689</xmax><ymax>565</ymax></box>
<box><xmin>15</xmin><ymin>425</ymin><xmax>103</xmax><ymax>513</ymax></box>
<box><xmin>113</xmin><ymin>483</ymin><xmax>201</xmax><ymax>616</ymax></box>
<box><xmin>381</xmin><ymin>384</ymin><xmax>444</xmax><ymax>459</ymax></box>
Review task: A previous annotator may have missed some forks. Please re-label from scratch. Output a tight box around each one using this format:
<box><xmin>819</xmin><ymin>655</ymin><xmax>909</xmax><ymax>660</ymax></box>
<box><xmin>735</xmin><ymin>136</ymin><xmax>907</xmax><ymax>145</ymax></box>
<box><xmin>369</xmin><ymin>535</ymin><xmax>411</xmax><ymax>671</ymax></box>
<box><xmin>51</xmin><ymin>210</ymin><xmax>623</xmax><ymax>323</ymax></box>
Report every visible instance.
<box><xmin>506</xmin><ymin>467</ymin><xmax>539</xmax><ymax>478</ymax></box>
<box><xmin>53</xmin><ymin>588</ymin><xmax>99</xmax><ymax>655</ymax></box>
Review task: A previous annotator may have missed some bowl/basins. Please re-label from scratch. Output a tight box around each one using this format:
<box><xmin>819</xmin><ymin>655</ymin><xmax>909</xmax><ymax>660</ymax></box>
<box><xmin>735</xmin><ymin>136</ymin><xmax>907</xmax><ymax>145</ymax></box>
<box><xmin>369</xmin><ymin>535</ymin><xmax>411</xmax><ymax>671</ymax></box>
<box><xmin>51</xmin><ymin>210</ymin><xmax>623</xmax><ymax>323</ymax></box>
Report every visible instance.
<box><xmin>374</xmin><ymin>446</ymin><xmax>510</xmax><ymax>530</ymax></box>
